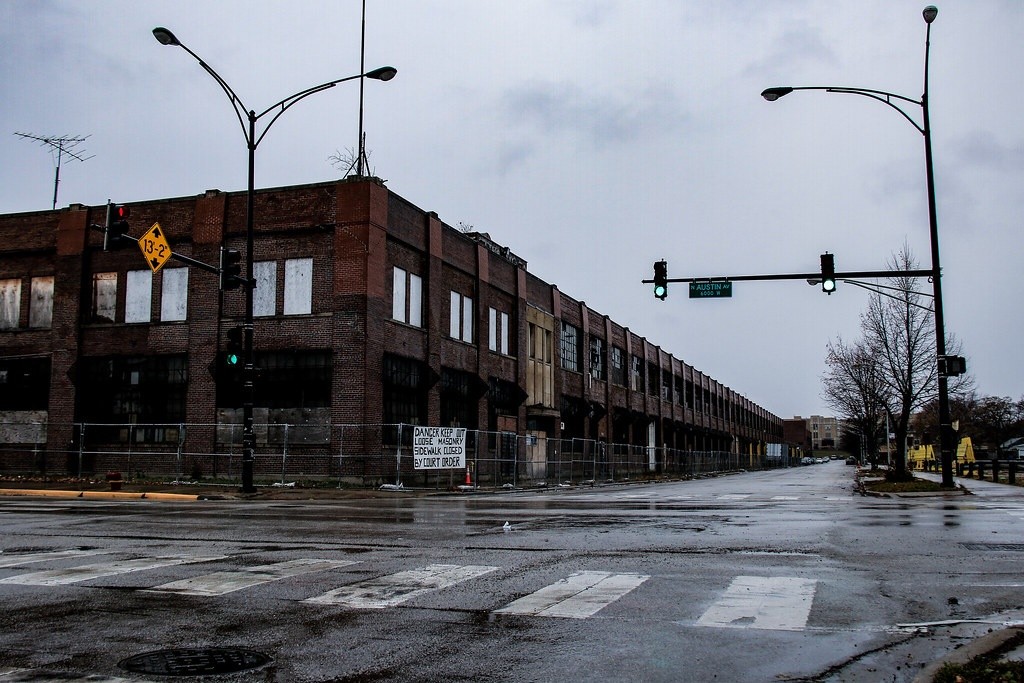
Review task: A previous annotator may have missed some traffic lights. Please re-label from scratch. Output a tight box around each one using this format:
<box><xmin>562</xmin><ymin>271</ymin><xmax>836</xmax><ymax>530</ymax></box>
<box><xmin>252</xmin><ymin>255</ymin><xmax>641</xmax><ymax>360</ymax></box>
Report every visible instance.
<box><xmin>216</xmin><ymin>244</ymin><xmax>243</xmax><ymax>291</ymax></box>
<box><xmin>103</xmin><ymin>198</ymin><xmax>133</xmax><ymax>253</ymax></box>
<box><xmin>948</xmin><ymin>356</ymin><xmax>967</xmax><ymax>373</ymax></box>
<box><xmin>226</xmin><ymin>327</ymin><xmax>244</xmax><ymax>368</ymax></box>
<box><xmin>820</xmin><ymin>253</ymin><xmax>837</xmax><ymax>293</ymax></box>
<box><xmin>653</xmin><ymin>261</ymin><xmax>668</xmax><ymax>302</ymax></box>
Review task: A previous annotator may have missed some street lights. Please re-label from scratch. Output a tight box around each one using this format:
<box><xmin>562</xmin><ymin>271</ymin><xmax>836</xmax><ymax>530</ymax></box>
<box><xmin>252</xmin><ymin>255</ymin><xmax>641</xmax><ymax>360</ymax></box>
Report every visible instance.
<box><xmin>759</xmin><ymin>4</ymin><xmax>955</xmax><ymax>485</ymax></box>
<box><xmin>152</xmin><ymin>25</ymin><xmax>400</xmax><ymax>492</ymax></box>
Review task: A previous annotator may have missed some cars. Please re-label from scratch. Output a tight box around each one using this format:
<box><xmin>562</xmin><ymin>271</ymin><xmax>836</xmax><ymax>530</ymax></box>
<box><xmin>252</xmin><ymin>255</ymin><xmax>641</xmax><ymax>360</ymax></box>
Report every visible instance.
<box><xmin>846</xmin><ymin>457</ymin><xmax>856</xmax><ymax>465</ymax></box>
<box><xmin>830</xmin><ymin>455</ymin><xmax>837</xmax><ymax>460</ymax></box>
<box><xmin>801</xmin><ymin>456</ymin><xmax>830</xmax><ymax>465</ymax></box>
<box><xmin>839</xmin><ymin>455</ymin><xmax>845</xmax><ymax>459</ymax></box>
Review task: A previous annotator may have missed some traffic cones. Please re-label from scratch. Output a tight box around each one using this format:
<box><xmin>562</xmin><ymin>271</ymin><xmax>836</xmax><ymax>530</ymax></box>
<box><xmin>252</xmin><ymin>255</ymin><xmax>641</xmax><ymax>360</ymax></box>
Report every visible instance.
<box><xmin>464</xmin><ymin>465</ymin><xmax>473</xmax><ymax>486</ymax></box>
<box><xmin>858</xmin><ymin>460</ymin><xmax>860</xmax><ymax>468</ymax></box>
